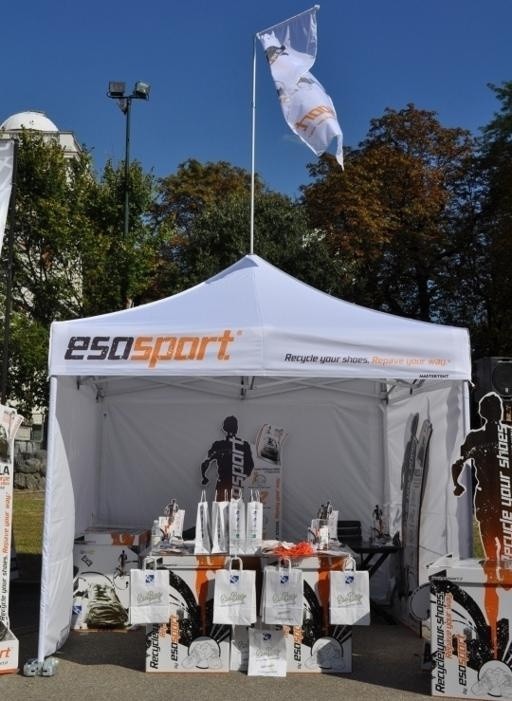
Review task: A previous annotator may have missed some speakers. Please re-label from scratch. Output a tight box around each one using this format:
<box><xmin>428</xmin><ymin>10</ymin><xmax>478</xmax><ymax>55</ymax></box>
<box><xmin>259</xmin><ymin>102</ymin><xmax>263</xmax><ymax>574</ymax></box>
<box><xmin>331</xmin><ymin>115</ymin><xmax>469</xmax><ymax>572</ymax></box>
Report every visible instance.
<box><xmin>473</xmin><ymin>356</ymin><xmax>512</xmax><ymax>403</ymax></box>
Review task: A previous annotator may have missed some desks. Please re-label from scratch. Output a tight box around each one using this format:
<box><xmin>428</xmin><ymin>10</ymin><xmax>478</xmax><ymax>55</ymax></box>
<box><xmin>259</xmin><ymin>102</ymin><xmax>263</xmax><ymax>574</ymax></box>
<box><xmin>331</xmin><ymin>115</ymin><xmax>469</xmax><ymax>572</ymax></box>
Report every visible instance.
<box><xmin>346</xmin><ymin>538</ymin><xmax>404</xmax><ymax>628</ymax></box>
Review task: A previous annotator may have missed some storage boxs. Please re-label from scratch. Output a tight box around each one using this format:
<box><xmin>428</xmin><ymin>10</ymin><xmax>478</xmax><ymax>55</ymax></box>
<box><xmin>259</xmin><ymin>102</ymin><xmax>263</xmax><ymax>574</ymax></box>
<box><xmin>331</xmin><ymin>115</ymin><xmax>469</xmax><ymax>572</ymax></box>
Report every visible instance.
<box><xmin>73</xmin><ymin>538</ymin><xmax>353</xmax><ymax>674</ymax></box>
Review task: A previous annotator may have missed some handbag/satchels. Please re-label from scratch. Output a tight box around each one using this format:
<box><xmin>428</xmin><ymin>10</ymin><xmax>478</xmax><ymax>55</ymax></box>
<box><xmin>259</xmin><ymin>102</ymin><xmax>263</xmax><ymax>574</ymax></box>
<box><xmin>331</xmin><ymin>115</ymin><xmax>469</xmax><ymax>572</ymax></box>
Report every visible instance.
<box><xmin>244</xmin><ymin>490</ymin><xmax>263</xmax><ymax>558</ymax></box>
<box><xmin>261</xmin><ymin>557</ymin><xmax>304</xmax><ymax>628</ymax></box>
<box><xmin>330</xmin><ymin>557</ymin><xmax>373</xmax><ymax>629</ymax></box>
<box><xmin>210</xmin><ymin>558</ymin><xmax>258</xmax><ymax>629</ymax></box>
<box><xmin>245</xmin><ymin>625</ymin><xmax>287</xmax><ymax>679</ymax></box>
<box><xmin>227</xmin><ymin>485</ymin><xmax>247</xmax><ymax>558</ymax></box>
<box><xmin>193</xmin><ymin>490</ymin><xmax>211</xmax><ymax>558</ymax></box>
<box><xmin>210</xmin><ymin>489</ymin><xmax>230</xmax><ymax>558</ymax></box>
<box><xmin>124</xmin><ymin>556</ymin><xmax>172</xmax><ymax>627</ymax></box>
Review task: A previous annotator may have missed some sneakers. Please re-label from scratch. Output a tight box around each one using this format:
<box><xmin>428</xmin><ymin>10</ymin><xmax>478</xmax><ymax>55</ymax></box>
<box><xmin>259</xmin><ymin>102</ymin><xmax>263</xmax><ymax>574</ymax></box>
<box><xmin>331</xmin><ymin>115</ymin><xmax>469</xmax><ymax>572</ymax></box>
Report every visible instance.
<box><xmin>83</xmin><ymin>581</ymin><xmax>129</xmax><ymax>630</ymax></box>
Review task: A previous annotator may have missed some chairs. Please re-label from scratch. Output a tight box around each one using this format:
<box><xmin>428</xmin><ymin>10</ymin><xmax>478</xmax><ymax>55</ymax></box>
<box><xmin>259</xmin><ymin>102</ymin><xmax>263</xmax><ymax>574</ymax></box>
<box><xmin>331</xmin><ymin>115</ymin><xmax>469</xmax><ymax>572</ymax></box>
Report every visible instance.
<box><xmin>336</xmin><ymin>521</ymin><xmax>377</xmax><ymax>612</ymax></box>
